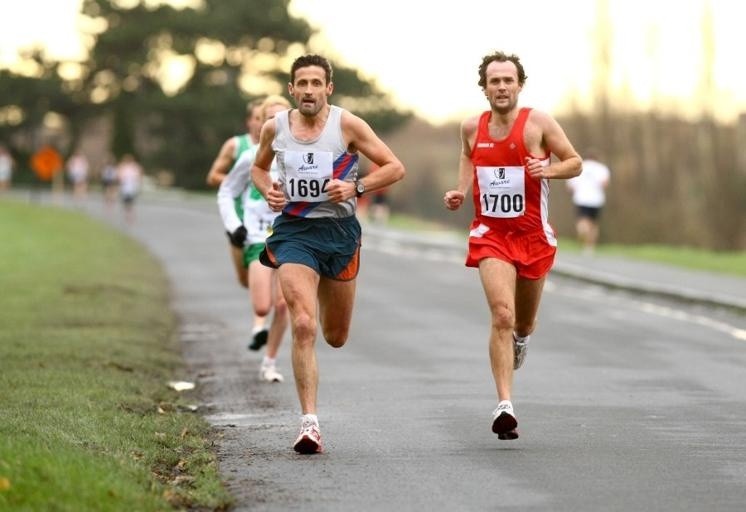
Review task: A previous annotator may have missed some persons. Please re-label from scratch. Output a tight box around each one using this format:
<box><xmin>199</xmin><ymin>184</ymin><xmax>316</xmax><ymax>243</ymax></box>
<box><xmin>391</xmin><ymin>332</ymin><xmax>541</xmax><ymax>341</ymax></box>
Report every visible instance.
<box><xmin>369</xmin><ymin>161</ymin><xmax>391</xmax><ymax>222</ymax></box>
<box><xmin>565</xmin><ymin>147</ymin><xmax>612</xmax><ymax>251</ymax></box>
<box><xmin>357</xmin><ymin>167</ymin><xmax>370</xmax><ymax>217</ymax></box>
<box><xmin>443</xmin><ymin>51</ymin><xmax>585</xmax><ymax>441</ymax></box>
<box><xmin>251</xmin><ymin>53</ymin><xmax>407</xmax><ymax>455</ymax></box>
<box><xmin>215</xmin><ymin>94</ymin><xmax>294</xmax><ymax>384</ymax></box>
<box><xmin>206</xmin><ymin>97</ymin><xmax>267</xmax><ymax>351</ymax></box>
<box><xmin>0</xmin><ymin>141</ymin><xmax>146</xmax><ymax>223</ymax></box>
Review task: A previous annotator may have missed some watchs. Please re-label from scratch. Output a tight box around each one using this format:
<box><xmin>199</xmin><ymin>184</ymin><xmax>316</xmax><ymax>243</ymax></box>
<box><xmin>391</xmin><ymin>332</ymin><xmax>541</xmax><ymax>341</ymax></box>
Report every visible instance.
<box><xmin>353</xmin><ymin>179</ymin><xmax>365</xmax><ymax>198</ymax></box>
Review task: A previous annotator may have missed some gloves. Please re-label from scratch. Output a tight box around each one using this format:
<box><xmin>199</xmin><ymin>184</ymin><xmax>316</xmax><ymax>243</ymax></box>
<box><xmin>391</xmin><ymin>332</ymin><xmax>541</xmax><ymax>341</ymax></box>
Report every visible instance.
<box><xmin>230</xmin><ymin>227</ymin><xmax>247</xmax><ymax>247</ymax></box>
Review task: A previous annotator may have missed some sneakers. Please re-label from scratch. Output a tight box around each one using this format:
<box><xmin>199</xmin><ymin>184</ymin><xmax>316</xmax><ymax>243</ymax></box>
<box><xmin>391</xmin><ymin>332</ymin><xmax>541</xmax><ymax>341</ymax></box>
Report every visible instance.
<box><xmin>491</xmin><ymin>406</ymin><xmax>519</xmax><ymax>440</ymax></box>
<box><xmin>513</xmin><ymin>339</ymin><xmax>529</xmax><ymax>370</ymax></box>
<box><xmin>248</xmin><ymin>325</ymin><xmax>269</xmax><ymax>350</ymax></box>
<box><xmin>256</xmin><ymin>363</ymin><xmax>282</xmax><ymax>382</ymax></box>
<box><xmin>293</xmin><ymin>423</ymin><xmax>325</xmax><ymax>454</ymax></box>
